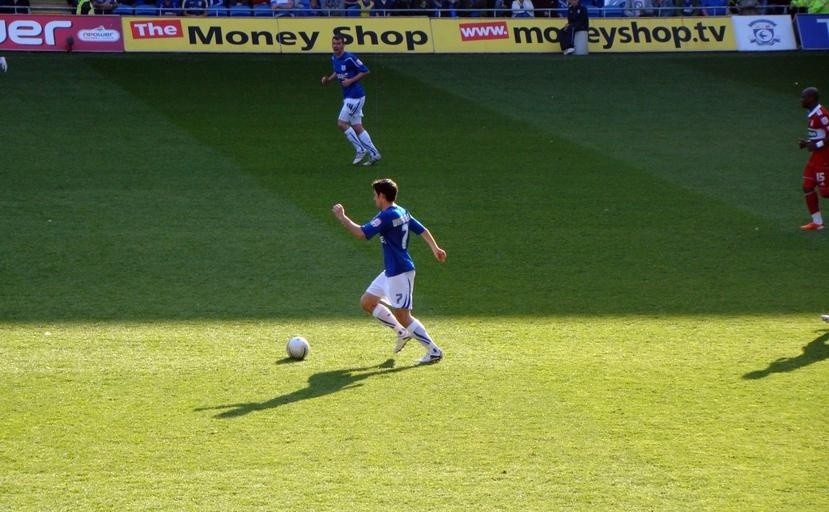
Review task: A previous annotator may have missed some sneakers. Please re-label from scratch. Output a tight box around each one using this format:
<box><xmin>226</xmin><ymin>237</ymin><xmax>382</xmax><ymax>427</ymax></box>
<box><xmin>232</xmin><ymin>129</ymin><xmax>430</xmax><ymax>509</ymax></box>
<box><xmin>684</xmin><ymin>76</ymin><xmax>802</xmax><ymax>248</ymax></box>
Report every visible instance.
<box><xmin>353</xmin><ymin>148</ymin><xmax>381</xmax><ymax>166</ymax></box>
<box><xmin>800</xmin><ymin>221</ymin><xmax>824</xmax><ymax>231</ymax></box>
<box><xmin>420</xmin><ymin>349</ymin><xmax>443</xmax><ymax>363</ymax></box>
<box><xmin>394</xmin><ymin>329</ymin><xmax>412</xmax><ymax>352</ymax></box>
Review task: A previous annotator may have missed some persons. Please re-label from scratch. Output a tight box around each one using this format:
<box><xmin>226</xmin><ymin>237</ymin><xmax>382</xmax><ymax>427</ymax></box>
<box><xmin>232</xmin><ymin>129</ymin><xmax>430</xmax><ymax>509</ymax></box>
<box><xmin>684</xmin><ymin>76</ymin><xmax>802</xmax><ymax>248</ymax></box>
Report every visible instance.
<box><xmin>799</xmin><ymin>86</ymin><xmax>829</xmax><ymax>232</ymax></box>
<box><xmin>66</xmin><ymin>1</ymin><xmax>829</xmax><ymax>20</ymax></box>
<box><xmin>558</xmin><ymin>1</ymin><xmax>590</xmax><ymax>58</ymax></box>
<box><xmin>1</xmin><ymin>56</ymin><xmax>9</xmax><ymax>75</ymax></box>
<box><xmin>321</xmin><ymin>35</ymin><xmax>382</xmax><ymax>168</ymax></box>
<box><xmin>332</xmin><ymin>179</ymin><xmax>450</xmax><ymax>368</ymax></box>
<box><xmin>0</xmin><ymin>1</ymin><xmax>30</xmax><ymax>14</ymax></box>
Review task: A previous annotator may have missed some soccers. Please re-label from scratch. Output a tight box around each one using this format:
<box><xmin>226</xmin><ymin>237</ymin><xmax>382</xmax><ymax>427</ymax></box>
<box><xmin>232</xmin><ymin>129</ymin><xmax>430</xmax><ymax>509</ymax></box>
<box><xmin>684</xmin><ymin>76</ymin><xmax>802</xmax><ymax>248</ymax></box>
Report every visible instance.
<box><xmin>286</xmin><ymin>336</ymin><xmax>310</xmax><ymax>360</ymax></box>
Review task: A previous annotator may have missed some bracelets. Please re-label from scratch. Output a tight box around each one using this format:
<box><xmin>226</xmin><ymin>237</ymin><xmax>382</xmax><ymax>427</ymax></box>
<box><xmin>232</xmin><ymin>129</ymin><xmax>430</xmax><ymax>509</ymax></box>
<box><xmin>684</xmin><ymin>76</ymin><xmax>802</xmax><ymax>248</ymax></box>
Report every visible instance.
<box><xmin>815</xmin><ymin>140</ymin><xmax>826</xmax><ymax>151</ymax></box>
